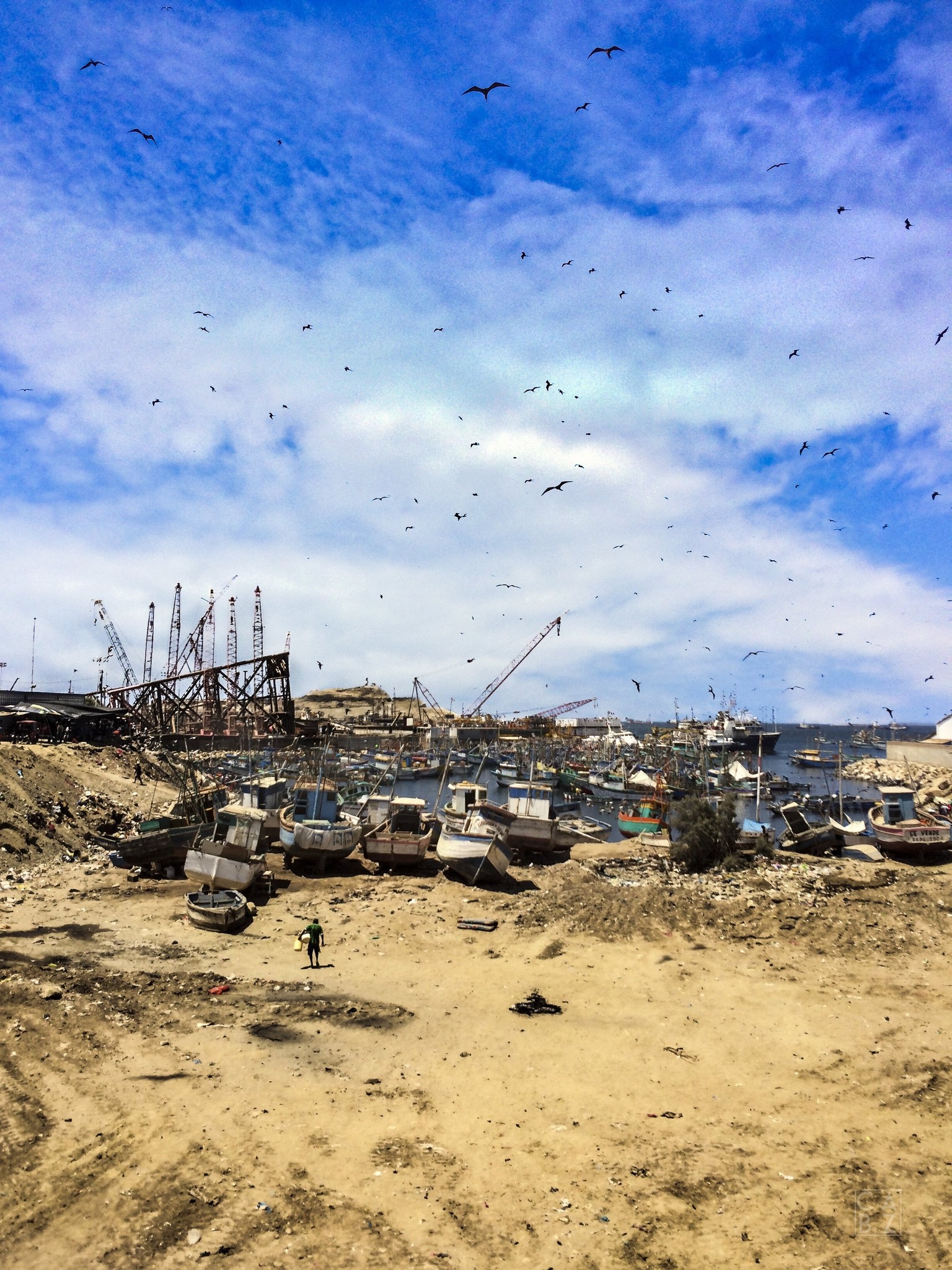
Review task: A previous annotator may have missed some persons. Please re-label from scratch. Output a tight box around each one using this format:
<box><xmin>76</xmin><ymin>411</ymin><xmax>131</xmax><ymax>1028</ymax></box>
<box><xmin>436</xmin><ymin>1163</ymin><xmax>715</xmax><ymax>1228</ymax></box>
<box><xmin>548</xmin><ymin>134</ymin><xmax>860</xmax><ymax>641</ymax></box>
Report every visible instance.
<box><xmin>133</xmin><ymin>764</ymin><xmax>143</xmax><ymax>785</ymax></box>
<box><xmin>90</xmin><ymin>719</ymin><xmax>97</xmax><ymax>740</ymax></box>
<box><xmin>56</xmin><ymin>721</ymin><xmax>64</xmax><ymax>743</ymax></box>
<box><xmin>298</xmin><ymin>918</ymin><xmax>324</xmax><ymax>968</ymax></box>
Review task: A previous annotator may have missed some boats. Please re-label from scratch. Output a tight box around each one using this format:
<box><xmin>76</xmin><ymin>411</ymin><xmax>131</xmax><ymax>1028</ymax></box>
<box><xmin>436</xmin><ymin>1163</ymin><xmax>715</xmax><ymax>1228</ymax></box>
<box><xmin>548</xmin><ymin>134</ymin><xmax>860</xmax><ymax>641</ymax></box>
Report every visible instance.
<box><xmin>788</xmin><ymin>748</ymin><xmax>855</xmax><ymax>768</ymax></box>
<box><xmin>435</xmin><ymin>740</ymin><xmax>520</xmax><ymax>887</ymax></box>
<box><xmin>279</xmin><ymin>742</ymin><xmax>361</xmax><ymax>868</ymax></box>
<box><xmin>867</xmin><ymin>754</ymin><xmax>952</xmax><ymax>855</ymax></box>
<box><xmin>183</xmin><ymin>889</ymin><xmax>248</xmax><ymax>933</ymax></box>
<box><xmin>355</xmin><ymin>743</ymin><xmax>453</xmax><ymax>867</ymax></box>
<box><xmin>184</xmin><ymin>716</ymin><xmax>269</xmax><ymax>892</ymax></box>
<box><xmin>90</xmin><ymin>688</ymin><xmax>952</xmax><ymax>893</ymax></box>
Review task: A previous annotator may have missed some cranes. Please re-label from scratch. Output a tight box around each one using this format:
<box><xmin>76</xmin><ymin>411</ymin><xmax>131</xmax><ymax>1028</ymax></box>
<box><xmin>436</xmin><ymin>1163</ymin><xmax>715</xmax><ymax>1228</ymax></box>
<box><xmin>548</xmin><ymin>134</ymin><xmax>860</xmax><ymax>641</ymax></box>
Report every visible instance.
<box><xmin>93</xmin><ymin>574</ymin><xmax>291</xmax><ymax>736</ymax></box>
<box><xmin>412</xmin><ymin>616</ymin><xmax>597</xmax><ymax>719</ymax></box>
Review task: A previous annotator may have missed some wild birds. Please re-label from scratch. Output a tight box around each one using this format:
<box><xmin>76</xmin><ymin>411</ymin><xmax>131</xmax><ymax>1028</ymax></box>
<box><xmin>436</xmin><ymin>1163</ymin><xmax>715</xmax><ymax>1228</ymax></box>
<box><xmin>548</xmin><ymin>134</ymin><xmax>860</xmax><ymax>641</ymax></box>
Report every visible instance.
<box><xmin>461</xmin><ymin>82</ymin><xmax>511</xmax><ymax>102</ymax></box>
<box><xmin>905</xmin><ymin>218</ymin><xmax>915</xmax><ymax>230</ymax></box>
<box><xmin>665</xmin><ymin>287</ymin><xmax>672</xmax><ymax>293</ymax></box>
<box><xmin>79</xmin><ymin>57</ymin><xmax>109</xmax><ymax>70</ymax></box>
<box><xmin>16</xmin><ymin>309</ymin><xmax>951</xmax><ymax>798</ymax></box>
<box><xmin>521</xmin><ymin>252</ymin><xmax>529</xmax><ymax>259</ymax></box>
<box><xmin>837</xmin><ymin>206</ymin><xmax>852</xmax><ymax>215</ymax></box>
<box><xmin>575</xmin><ymin>102</ymin><xmax>591</xmax><ymax>113</ymax></box>
<box><xmin>160</xmin><ymin>6</ymin><xmax>173</xmax><ymax>11</ymax></box>
<box><xmin>652</xmin><ymin>309</ymin><xmax>659</xmax><ymax>312</ymax></box>
<box><xmin>275</xmin><ymin>140</ymin><xmax>282</xmax><ymax>146</ymax></box>
<box><xmin>588</xmin><ymin>46</ymin><xmax>625</xmax><ymax>60</ymax></box>
<box><xmin>589</xmin><ymin>268</ymin><xmax>597</xmax><ymax>274</ymax></box>
<box><xmin>561</xmin><ymin>260</ymin><xmax>574</xmax><ymax>267</ymax></box>
<box><xmin>789</xmin><ymin>349</ymin><xmax>800</xmax><ymax>359</ymax></box>
<box><xmin>934</xmin><ymin>325</ymin><xmax>949</xmax><ymax>345</ymax></box>
<box><xmin>126</xmin><ymin>128</ymin><xmax>158</xmax><ymax>148</ymax></box>
<box><xmin>619</xmin><ymin>290</ymin><xmax>628</xmax><ymax>299</ymax></box>
<box><xmin>699</xmin><ymin>314</ymin><xmax>703</xmax><ymax>318</ymax></box>
<box><xmin>767</xmin><ymin>163</ymin><xmax>789</xmax><ymax>171</ymax></box>
<box><xmin>854</xmin><ymin>256</ymin><xmax>875</xmax><ymax>261</ymax></box>
